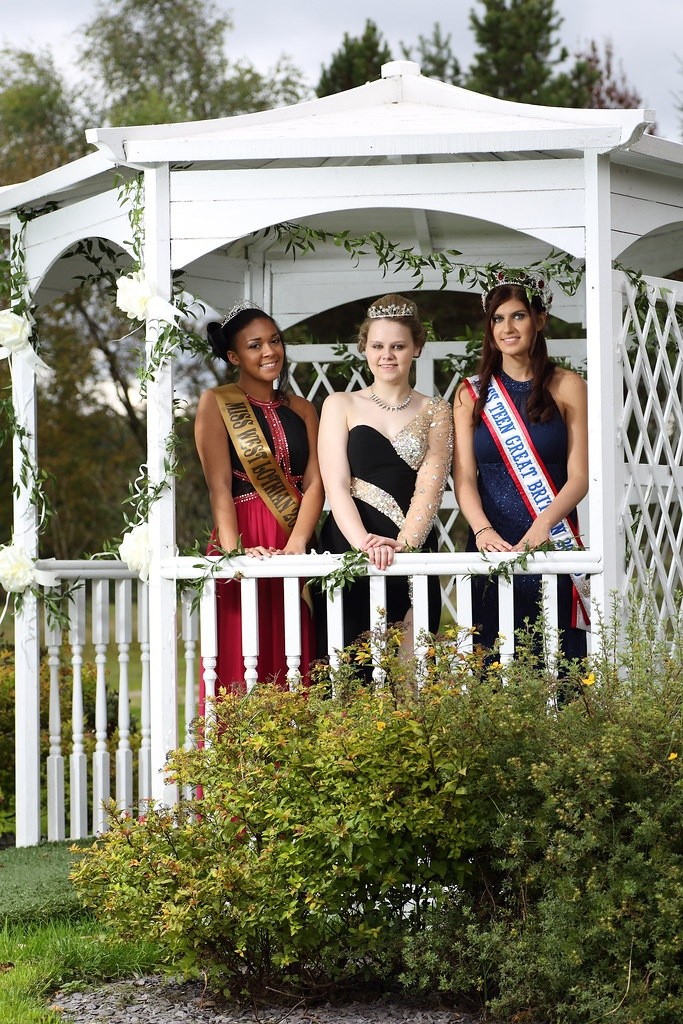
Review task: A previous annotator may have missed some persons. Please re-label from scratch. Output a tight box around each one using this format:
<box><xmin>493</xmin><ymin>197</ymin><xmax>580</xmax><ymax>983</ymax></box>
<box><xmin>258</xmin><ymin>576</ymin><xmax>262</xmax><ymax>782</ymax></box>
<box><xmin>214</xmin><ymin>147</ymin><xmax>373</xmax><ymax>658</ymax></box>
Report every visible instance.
<box><xmin>196</xmin><ymin>300</ymin><xmax>326</xmax><ymax>850</ymax></box>
<box><xmin>318</xmin><ymin>295</ymin><xmax>453</xmax><ymax>704</ymax></box>
<box><xmin>455</xmin><ymin>269</ymin><xmax>589</xmax><ymax>711</ymax></box>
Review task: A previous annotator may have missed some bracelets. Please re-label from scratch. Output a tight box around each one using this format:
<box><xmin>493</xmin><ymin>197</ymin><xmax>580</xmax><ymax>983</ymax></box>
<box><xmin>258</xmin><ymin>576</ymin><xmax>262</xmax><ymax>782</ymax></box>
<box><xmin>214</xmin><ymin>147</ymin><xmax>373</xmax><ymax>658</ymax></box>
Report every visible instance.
<box><xmin>475</xmin><ymin>527</ymin><xmax>494</xmax><ymax>537</ymax></box>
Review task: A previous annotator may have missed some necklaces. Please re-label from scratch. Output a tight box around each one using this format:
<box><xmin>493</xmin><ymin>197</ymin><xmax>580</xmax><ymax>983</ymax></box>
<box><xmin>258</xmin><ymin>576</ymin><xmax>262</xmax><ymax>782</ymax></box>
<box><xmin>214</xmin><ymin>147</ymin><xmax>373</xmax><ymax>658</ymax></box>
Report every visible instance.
<box><xmin>371</xmin><ymin>385</ymin><xmax>412</xmax><ymax>412</ymax></box>
